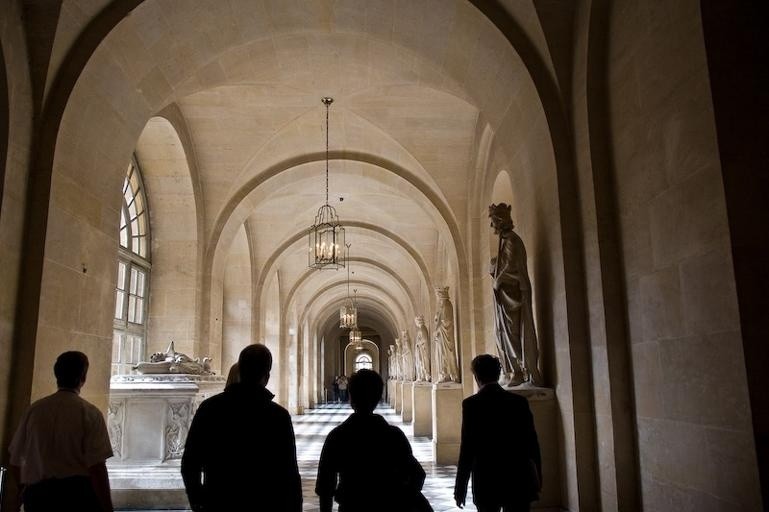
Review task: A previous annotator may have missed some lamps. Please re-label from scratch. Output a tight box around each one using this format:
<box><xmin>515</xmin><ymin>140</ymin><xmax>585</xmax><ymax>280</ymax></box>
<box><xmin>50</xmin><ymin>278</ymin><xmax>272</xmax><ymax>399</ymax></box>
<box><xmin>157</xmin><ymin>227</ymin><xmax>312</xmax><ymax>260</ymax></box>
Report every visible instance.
<box><xmin>339</xmin><ymin>243</ymin><xmax>359</xmax><ymax>330</ymax></box>
<box><xmin>308</xmin><ymin>97</ymin><xmax>348</xmax><ymax>274</ymax></box>
<box><xmin>349</xmin><ymin>288</ymin><xmax>363</xmax><ymax>344</ymax></box>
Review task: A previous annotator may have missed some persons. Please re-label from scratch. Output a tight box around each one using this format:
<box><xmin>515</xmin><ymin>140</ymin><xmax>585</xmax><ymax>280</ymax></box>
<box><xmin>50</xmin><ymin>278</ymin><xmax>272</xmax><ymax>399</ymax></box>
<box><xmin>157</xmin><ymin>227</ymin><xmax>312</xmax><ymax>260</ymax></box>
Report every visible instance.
<box><xmin>453</xmin><ymin>354</ymin><xmax>542</xmax><ymax>512</ymax></box>
<box><xmin>0</xmin><ymin>350</ymin><xmax>114</xmax><ymax>512</ymax></box>
<box><xmin>487</xmin><ymin>202</ymin><xmax>542</xmax><ymax>388</ymax></box>
<box><xmin>386</xmin><ymin>314</ymin><xmax>432</xmax><ymax>382</ymax></box>
<box><xmin>431</xmin><ymin>286</ymin><xmax>461</xmax><ymax>384</ymax></box>
<box><xmin>225</xmin><ymin>362</ymin><xmax>241</xmax><ymax>387</ymax></box>
<box><xmin>331</xmin><ymin>375</ymin><xmax>349</xmax><ymax>403</ymax></box>
<box><xmin>315</xmin><ymin>368</ymin><xmax>434</xmax><ymax>512</ymax></box>
<box><xmin>181</xmin><ymin>344</ymin><xmax>303</xmax><ymax>511</ymax></box>
